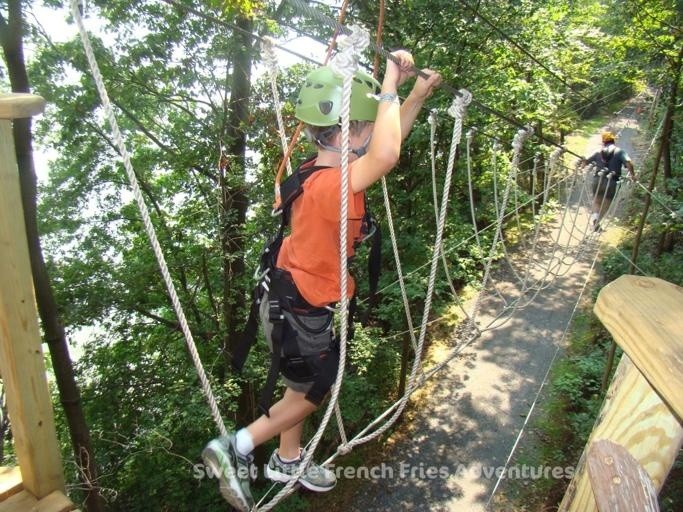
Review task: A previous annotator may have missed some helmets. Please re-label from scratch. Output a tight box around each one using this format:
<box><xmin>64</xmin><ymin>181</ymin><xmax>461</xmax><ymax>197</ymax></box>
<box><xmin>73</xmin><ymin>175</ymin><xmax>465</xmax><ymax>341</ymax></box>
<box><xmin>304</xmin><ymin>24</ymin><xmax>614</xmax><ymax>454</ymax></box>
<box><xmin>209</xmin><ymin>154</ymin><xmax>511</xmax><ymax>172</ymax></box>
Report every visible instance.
<box><xmin>294</xmin><ymin>66</ymin><xmax>381</xmax><ymax>127</ymax></box>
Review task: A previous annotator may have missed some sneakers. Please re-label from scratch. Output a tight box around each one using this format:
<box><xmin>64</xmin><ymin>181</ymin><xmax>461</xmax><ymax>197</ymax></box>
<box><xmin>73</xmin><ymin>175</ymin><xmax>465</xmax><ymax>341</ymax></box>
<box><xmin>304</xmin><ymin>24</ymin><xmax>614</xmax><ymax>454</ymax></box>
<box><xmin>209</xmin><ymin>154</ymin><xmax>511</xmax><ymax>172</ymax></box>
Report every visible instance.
<box><xmin>592</xmin><ymin>218</ymin><xmax>601</xmax><ymax>231</ymax></box>
<box><xmin>266</xmin><ymin>447</ymin><xmax>337</xmax><ymax>492</ymax></box>
<box><xmin>201</xmin><ymin>433</ymin><xmax>255</xmax><ymax>512</ymax></box>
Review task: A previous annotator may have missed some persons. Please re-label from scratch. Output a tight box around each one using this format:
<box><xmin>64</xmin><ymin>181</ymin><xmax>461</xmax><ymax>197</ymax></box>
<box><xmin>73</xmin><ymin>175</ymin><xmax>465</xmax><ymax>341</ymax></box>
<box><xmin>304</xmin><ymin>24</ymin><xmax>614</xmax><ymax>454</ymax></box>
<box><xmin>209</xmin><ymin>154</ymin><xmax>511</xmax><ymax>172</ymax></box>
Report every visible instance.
<box><xmin>200</xmin><ymin>50</ymin><xmax>442</xmax><ymax>512</ymax></box>
<box><xmin>575</xmin><ymin>131</ymin><xmax>637</xmax><ymax>234</ymax></box>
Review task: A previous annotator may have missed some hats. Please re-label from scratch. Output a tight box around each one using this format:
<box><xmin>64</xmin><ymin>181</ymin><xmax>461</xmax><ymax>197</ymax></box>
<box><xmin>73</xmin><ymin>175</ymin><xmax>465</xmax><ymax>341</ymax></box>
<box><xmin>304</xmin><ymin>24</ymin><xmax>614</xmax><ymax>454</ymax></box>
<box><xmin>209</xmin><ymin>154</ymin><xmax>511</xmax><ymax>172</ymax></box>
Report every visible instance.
<box><xmin>601</xmin><ymin>131</ymin><xmax>614</xmax><ymax>142</ymax></box>
